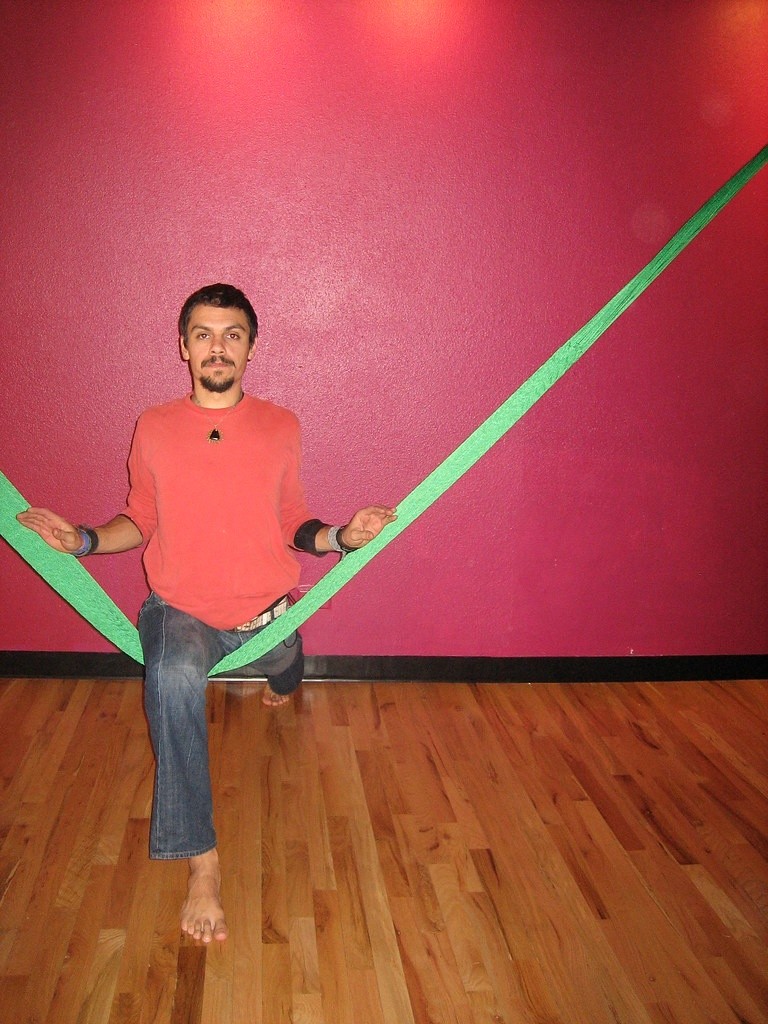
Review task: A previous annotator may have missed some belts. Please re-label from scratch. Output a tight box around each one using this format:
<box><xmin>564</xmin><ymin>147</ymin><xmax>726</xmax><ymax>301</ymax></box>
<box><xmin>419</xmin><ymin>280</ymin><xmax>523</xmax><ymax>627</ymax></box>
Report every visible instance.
<box><xmin>225</xmin><ymin>596</ymin><xmax>290</xmax><ymax>634</ymax></box>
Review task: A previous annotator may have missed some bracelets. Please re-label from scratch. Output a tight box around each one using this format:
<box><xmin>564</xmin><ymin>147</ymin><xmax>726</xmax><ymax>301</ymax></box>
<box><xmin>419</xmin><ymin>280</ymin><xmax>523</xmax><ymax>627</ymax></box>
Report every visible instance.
<box><xmin>73</xmin><ymin>523</ymin><xmax>99</xmax><ymax>558</ymax></box>
<box><xmin>328</xmin><ymin>524</ymin><xmax>358</xmax><ymax>554</ymax></box>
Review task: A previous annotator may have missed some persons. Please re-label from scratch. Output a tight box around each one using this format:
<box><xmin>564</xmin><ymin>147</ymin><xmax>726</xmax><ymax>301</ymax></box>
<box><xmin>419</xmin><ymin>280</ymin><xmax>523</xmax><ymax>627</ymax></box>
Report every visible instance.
<box><xmin>14</xmin><ymin>284</ymin><xmax>399</xmax><ymax>943</ymax></box>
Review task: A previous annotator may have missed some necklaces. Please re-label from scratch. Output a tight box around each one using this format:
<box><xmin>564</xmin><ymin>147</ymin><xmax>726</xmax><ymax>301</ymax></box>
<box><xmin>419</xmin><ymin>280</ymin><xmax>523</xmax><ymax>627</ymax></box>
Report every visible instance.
<box><xmin>192</xmin><ymin>389</ymin><xmax>243</xmax><ymax>444</ymax></box>
